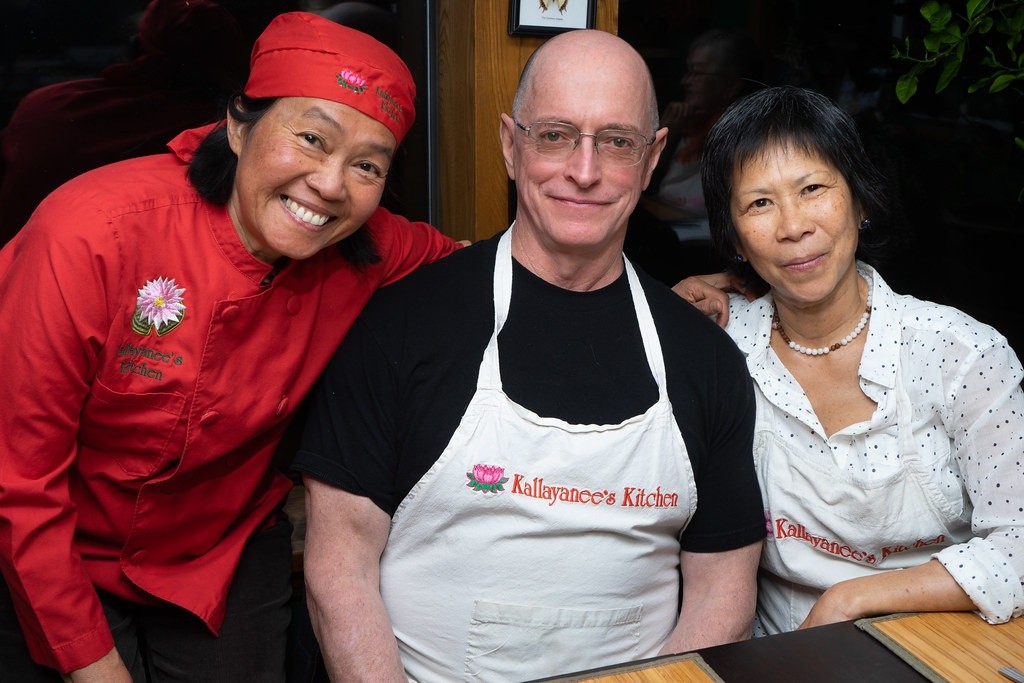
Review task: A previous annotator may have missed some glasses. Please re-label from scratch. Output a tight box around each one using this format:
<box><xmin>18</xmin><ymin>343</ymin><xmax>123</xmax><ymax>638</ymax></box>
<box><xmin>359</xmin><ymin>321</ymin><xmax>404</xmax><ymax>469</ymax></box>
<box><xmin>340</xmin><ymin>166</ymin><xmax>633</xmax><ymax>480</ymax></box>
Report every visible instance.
<box><xmin>510</xmin><ymin>115</ymin><xmax>657</xmax><ymax>167</ymax></box>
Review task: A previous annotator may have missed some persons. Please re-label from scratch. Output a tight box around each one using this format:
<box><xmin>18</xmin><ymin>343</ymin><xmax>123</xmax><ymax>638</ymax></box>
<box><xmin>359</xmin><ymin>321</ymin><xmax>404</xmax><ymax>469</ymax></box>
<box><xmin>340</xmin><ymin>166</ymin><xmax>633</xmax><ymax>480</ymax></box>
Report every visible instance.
<box><xmin>284</xmin><ymin>30</ymin><xmax>763</xmax><ymax>682</ymax></box>
<box><xmin>692</xmin><ymin>86</ymin><xmax>1024</xmax><ymax>641</ymax></box>
<box><xmin>2</xmin><ymin>11</ymin><xmax>756</xmax><ymax>681</ymax></box>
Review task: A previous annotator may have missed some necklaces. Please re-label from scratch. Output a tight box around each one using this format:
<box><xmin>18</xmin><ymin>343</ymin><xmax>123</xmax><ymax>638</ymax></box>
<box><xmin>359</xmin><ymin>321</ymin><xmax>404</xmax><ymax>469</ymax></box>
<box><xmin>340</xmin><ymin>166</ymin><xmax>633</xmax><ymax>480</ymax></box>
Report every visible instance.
<box><xmin>771</xmin><ymin>267</ymin><xmax>873</xmax><ymax>355</ymax></box>
